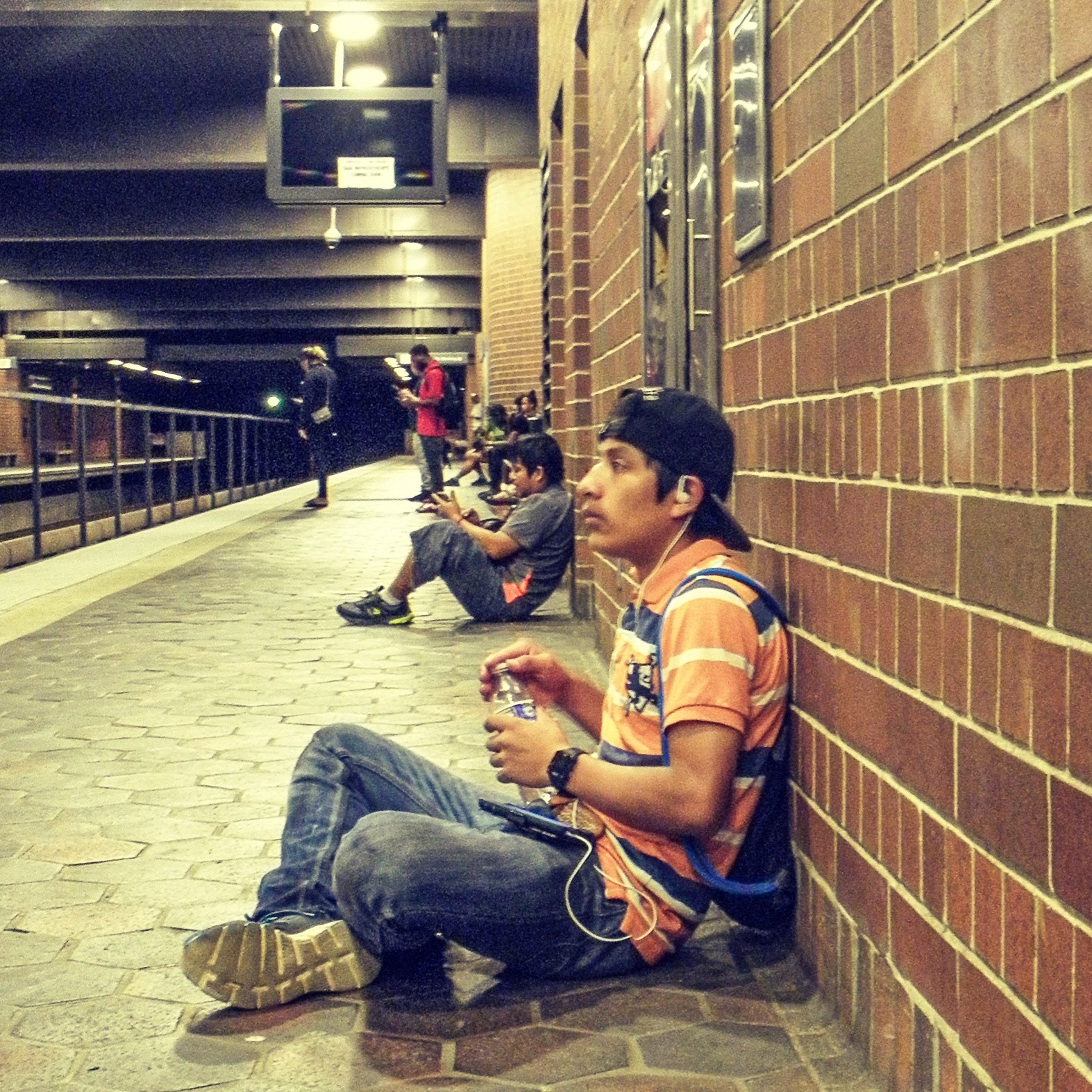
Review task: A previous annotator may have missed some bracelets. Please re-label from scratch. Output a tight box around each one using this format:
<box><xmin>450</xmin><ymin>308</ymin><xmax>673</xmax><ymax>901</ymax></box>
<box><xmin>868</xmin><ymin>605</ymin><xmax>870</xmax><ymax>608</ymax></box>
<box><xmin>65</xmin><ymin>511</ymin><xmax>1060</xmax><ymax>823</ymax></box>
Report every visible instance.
<box><xmin>456</xmin><ymin>518</ymin><xmax>464</xmax><ymax>525</ymax></box>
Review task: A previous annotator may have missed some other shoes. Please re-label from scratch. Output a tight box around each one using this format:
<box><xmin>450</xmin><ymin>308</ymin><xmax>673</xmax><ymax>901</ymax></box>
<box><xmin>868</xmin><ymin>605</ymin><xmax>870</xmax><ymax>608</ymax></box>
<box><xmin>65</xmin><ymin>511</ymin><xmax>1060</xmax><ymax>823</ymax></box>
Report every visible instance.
<box><xmin>472</xmin><ymin>478</ymin><xmax>488</xmax><ymax>486</ymax></box>
<box><xmin>443</xmin><ymin>478</ymin><xmax>460</xmax><ymax>487</ymax></box>
<box><xmin>478</xmin><ymin>487</ymin><xmax>501</xmax><ymax>500</ymax></box>
<box><xmin>180</xmin><ymin>914</ymin><xmax>381</xmax><ymax>1010</ymax></box>
<box><xmin>407</xmin><ymin>491</ymin><xmax>432</xmax><ymax>502</ymax></box>
<box><xmin>304</xmin><ymin>499</ymin><xmax>328</xmax><ymax>507</ymax></box>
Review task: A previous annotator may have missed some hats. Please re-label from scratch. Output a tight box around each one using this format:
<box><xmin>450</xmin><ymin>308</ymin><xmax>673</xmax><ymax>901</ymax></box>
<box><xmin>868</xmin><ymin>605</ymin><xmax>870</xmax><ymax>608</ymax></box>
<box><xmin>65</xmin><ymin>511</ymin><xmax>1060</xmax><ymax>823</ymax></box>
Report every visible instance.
<box><xmin>599</xmin><ymin>386</ymin><xmax>754</xmax><ymax>553</ymax></box>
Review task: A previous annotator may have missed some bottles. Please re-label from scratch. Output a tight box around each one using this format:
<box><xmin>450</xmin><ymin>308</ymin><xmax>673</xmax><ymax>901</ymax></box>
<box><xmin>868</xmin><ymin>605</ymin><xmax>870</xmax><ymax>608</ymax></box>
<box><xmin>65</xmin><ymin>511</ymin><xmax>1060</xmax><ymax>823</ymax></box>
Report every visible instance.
<box><xmin>489</xmin><ymin>660</ymin><xmax>560</xmax><ymax>805</ymax></box>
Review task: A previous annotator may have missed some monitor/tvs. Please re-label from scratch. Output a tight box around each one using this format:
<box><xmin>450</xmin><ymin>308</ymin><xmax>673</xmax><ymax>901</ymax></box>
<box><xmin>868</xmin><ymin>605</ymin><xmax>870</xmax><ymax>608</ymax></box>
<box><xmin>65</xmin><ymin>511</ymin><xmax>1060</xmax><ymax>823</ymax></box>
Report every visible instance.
<box><xmin>264</xmin><ymin>85</ymin><xmax>449</xmax><ymax>209</ymax></box>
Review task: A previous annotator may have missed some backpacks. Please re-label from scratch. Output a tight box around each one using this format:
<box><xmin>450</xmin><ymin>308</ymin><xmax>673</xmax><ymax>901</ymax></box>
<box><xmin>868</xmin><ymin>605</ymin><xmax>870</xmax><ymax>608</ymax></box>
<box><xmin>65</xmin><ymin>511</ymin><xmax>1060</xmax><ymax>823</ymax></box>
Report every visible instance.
<box><xmin>424</xmin><ymin>366</ymin><xmax>464</xmax><ymax>430</ymax></box>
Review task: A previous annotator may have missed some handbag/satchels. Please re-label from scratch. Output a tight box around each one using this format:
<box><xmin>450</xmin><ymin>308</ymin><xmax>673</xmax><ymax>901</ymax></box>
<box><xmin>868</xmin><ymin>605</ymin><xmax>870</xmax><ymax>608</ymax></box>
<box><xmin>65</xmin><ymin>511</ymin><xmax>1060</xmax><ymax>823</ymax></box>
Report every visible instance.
<box><xmin>310</xmin><ymin>406</ymin><xmax>332</xmax><ymax>425</ymax></box>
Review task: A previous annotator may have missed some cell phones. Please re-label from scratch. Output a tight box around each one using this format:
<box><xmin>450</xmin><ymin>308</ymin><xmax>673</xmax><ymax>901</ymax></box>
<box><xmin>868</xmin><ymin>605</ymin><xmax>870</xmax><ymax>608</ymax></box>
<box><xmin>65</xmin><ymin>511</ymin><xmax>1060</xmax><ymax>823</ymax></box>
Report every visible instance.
<box><xmin>437</xmin><ymin>493</ymin><xmax>450</xmax><ymax>503</ymax></box>
<box><xmin>480</xmin><ymin>799</ymin><xmax>594</xmax><ymax>847</ymax></box>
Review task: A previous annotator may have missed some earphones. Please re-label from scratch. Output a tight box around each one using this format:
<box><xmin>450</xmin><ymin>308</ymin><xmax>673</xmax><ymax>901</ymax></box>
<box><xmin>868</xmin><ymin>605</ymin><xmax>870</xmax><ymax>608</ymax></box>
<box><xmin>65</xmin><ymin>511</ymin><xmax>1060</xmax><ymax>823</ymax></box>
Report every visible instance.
<box><xmin>677</xmin><ymin>475</ymin><xmax>690</xmax><ymax>503</ymax></box>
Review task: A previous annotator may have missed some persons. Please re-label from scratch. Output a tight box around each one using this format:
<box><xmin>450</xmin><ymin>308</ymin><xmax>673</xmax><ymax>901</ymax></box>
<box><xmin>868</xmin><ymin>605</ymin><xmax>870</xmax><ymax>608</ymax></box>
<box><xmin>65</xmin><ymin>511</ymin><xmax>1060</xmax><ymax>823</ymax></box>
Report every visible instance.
<box><xmin>182</xmin><ymin>388</ymin><xmax>789</xmax><ymax>1010</ymax></box>
<box><xmin>337</xmin><ymin>342</ymin><xmax>574</xmax><ymax>627</ymax></box>
<box><xmin>299</xmin><ymin>346</ymin><xmax>332</xmax><ymax>508</ymax></box>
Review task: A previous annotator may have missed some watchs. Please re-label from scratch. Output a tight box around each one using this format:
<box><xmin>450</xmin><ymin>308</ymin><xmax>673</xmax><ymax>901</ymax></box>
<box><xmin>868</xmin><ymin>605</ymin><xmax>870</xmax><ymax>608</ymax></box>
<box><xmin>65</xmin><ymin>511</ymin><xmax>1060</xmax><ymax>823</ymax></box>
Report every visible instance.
<box><xmin>548</xmin><ymin>748</ymin><xmax>588</xmax><ymax>800</ymax></box>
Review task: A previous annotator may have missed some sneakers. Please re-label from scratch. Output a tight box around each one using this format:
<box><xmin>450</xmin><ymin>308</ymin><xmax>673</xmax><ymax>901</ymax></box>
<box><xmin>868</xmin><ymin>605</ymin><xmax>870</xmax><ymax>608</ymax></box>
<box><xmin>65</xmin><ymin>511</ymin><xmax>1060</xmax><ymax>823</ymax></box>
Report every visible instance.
<box><xmin>336</xmin><ymin>584</ymin><xmax>415</xmax><ymax>626</ymax></box>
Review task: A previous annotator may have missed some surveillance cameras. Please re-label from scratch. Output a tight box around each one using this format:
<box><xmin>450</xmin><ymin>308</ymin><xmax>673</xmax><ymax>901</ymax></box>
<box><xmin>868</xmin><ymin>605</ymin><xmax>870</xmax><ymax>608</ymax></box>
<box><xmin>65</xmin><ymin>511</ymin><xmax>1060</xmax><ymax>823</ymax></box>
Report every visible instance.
<box><xmin>324</xmin><ymin>228</ymin><xmax>341</xmax><ymax>249</ymax></box>
